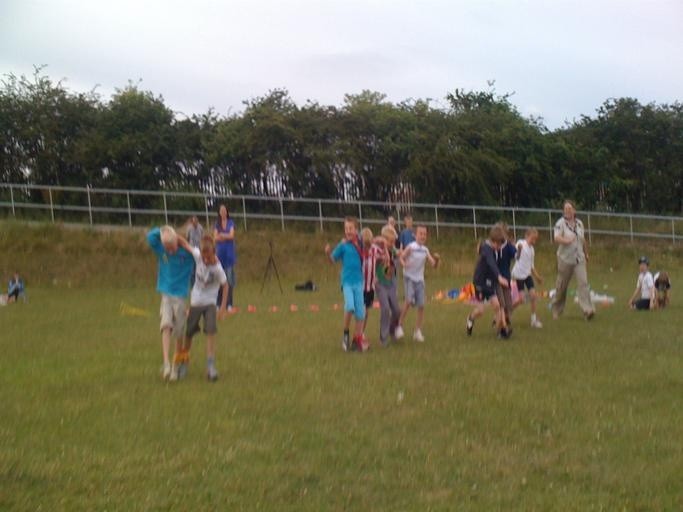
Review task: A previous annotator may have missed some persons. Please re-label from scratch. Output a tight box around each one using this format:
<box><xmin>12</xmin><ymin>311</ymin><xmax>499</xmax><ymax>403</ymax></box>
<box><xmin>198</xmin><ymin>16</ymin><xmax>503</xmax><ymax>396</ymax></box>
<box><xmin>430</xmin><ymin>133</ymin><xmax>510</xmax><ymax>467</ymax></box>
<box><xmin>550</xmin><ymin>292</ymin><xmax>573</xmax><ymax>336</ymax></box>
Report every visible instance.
<box><xmin>549</xmin><ymin>199</ymin><xmax>596</xmax><ymax>321</ymax></box>
<box><xmin>3</xmin><ymin>271</ymin><xmax>25</xmax><ymax>305</ymax></box>
<box><xmin>629</xmin><ymin>255</ymin><xmax>655</xmax><ymax>312</ymax></box>
<box><xmin>145</xmin><ymin>224</ymin><xmax>220</xmax><ymax>380</ymax></box>
<box><xmin>465</xmin><ymin>226</ymin><xmax>511</xmax><ymax>336</ymax></box>
<box><xmin>173</xmin><ymin>228</ymin><xmax>230</xmax><ymax>379</ymax></box>
<box><xmin>476</xmin><ymin>221</ymin><xmax>522</xmax><ymax>339</ymax></box>
<box><xmin>212</xmin><ymin>204</ymin><xmax>236</xmax><ymax>314</ymax></box>
<box><xmin>186</xmin><ymin>215</ymin><xmax>205</xmax><ymax>289</ymax></box>
<box><xmin>650</xmin><ymin>271</ymin><xmax>671</xmax><ymax>308</ymax></box>
<box><xmin>508</xmin><ymin>226</ymin><xmax>544</xmax><ymax>330</ymax></box>
<box><xmin>322</xmin><ymin>213</ymin><xmax>442</xmax><ymax>354</ymax></box>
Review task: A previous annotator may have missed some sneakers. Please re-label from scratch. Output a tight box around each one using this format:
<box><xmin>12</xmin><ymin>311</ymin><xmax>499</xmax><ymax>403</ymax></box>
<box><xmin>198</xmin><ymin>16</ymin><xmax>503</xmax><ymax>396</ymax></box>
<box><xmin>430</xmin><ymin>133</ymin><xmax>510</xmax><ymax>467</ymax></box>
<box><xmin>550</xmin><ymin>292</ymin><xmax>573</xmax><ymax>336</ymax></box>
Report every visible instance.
<box><xmin>530</xmin><ymin>319</ymin><xmax>543</xmax><ymax>330</ymax></box>
<box><xmin>208</xmin><ymin>358</ymin><xmax>217</xmax><ymax>381</ymax></box>
<box><xmin>493</xmin><ymin>319</ymin><xmax>512</xmax><ymax>338</ymax></box>
<box><xmin>342</xmin><ymin>324</ymin><xmax>425</xmax><ymax>352</ymax></box>
<box><xmin>467</xmin><ymin>313</ymin><xmax>473</xmax><ymax>335</ymax></box>
<box><xmin>160</xmin><ymin>352</ymin><xmax>188</xmax><ymax>381</ymax></box>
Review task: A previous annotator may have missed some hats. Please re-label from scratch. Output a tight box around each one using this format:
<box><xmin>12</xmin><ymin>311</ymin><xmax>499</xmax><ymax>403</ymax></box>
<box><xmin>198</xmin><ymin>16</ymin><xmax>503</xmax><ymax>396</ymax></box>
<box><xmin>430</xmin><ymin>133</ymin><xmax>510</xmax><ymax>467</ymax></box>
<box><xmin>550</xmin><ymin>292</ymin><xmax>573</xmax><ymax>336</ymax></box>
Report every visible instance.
<box><xmin>639</xmin><ymin>257</ymin><xmax>649</xmax><ymax>265</ymax></box>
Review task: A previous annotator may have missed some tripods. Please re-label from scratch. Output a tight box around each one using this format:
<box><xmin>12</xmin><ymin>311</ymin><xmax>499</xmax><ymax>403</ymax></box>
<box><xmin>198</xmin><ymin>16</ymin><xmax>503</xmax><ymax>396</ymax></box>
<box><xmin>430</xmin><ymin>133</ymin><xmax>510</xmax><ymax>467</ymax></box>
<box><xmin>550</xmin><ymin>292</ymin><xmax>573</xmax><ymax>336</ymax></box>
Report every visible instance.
<box><xmin>260</xmin><ymin>242</ymin><xmax>285</xmax><ymax>295</ymax></box>
